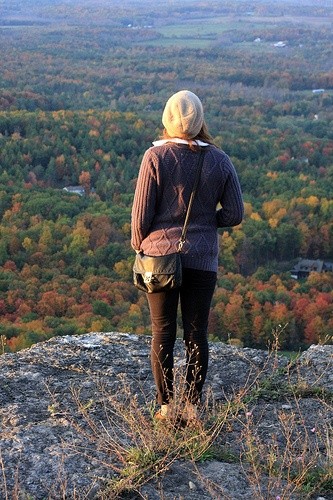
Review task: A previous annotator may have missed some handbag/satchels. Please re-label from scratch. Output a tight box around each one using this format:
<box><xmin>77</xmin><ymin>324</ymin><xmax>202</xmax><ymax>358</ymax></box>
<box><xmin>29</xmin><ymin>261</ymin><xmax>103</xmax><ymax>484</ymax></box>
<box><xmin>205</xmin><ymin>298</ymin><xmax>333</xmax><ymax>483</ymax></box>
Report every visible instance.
<box><xmin>132</xmin><ymin>251</ymin><xmax>181</xmax><ymax>292</ymax></box>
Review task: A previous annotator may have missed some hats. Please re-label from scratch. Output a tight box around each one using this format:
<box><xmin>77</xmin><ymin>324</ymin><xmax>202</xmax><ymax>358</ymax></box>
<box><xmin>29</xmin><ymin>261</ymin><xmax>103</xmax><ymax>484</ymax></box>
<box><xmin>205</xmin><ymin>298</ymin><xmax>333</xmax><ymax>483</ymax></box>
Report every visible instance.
<box><xmin>161</xmin><ymin>90</ymin><xmax>203</xmax><ymax>139</ymax></box>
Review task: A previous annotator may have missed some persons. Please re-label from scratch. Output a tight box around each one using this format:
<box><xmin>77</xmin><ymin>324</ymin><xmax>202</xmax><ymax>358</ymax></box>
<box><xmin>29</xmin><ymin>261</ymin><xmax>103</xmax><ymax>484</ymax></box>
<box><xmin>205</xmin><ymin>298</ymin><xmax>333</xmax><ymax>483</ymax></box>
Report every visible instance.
<box><xmin>129</xmin><ymin>89</ymin><xmax>244</xmax><ymax>422</ymax></box>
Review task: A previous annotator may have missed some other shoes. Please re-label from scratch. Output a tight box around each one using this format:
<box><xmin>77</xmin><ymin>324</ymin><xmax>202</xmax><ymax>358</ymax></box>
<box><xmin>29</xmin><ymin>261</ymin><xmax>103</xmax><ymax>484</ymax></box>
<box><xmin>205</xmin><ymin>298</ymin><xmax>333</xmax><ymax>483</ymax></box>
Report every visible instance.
<box><xmin>152</xmin><ymin>410</ymin><xmax>175</xmax><ymax>424</ymax></box>
